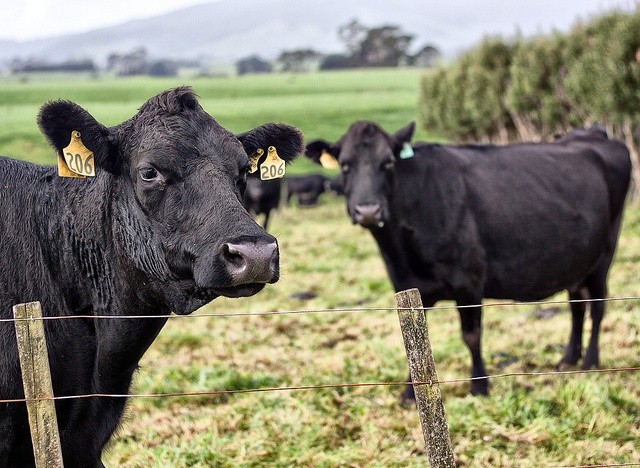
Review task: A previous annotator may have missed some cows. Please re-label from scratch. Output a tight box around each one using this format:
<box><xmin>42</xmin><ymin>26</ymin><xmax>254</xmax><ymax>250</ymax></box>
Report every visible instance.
<box><xmin>0</xmin><ymin>85</ymin><xmax>304</xmax><ymax>467</ymax></box>
<box><xmin>283</xmin><ymin>174</ymin><xmax>333</xmax><ymax>206</ymax></box>
<box><xmin>303</xmin><ymin>120</ymin><xmax>631</xmax><ymax>400</ymax></box>
<box><xmin>330</xmin><ymin>174</ymin><xmax>345</xmax><ymax>195</ymax></box>
<box><xmin>244</xmin><ymin>175</ymin><xmax>281</xmax><ymax>229</ymax></box>
